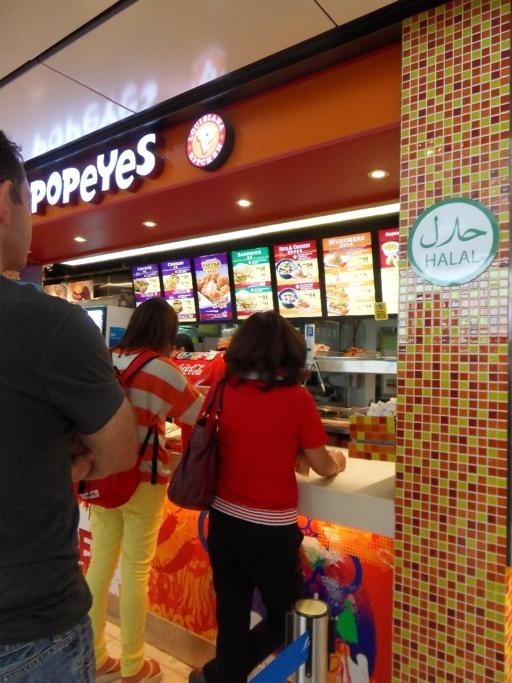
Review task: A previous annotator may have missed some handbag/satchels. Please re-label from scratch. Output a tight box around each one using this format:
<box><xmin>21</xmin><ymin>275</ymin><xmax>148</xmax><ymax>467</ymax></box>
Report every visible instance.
<box><xmin>166</xmin><ymin>416</ymin><xmax>219</xmax><ymax>510</ymax></box>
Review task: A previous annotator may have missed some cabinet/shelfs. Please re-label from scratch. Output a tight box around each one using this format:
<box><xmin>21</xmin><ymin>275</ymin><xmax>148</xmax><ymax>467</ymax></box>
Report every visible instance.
<box><xmin>301</xmin><ymin>349</ymin><xmax>397</xmax><ymax>438</ymax></box>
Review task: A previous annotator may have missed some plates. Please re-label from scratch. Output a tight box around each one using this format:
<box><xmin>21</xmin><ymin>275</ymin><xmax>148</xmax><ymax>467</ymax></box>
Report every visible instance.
<box><xmin>324</xmin><ymin>253</ymin><xmax>354</xmax><ymax>316</ymax></box>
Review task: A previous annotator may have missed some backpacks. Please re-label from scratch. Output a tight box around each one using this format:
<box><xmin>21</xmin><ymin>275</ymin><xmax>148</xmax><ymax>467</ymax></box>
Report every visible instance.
<box><xmin>73</xmin><ymin>350</ymin><xmax>161</xmax><ymax>508</ymax></box>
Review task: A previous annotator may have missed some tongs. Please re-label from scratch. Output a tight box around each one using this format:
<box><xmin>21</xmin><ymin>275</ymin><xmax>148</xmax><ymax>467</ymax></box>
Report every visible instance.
<box><xmin>303</xmin><ymin>360</ymin><xmax>327</xmax><ymax>393</ymax></box>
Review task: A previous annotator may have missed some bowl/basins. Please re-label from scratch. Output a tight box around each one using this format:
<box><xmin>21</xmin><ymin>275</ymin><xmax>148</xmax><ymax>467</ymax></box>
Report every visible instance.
<box><xmin>276</xmin><ymin>259</ymin><xmax>298</xmax><ymax>279</ymax></box>
<box><xmin>381</xmin><ymin>241</ymin><xmax>399</xmax><ymax>257</ymax></box>
<box><xmin>278</xmin><ymin>287</ymin><xmax>299</xmax><ymax>308</ymax></box>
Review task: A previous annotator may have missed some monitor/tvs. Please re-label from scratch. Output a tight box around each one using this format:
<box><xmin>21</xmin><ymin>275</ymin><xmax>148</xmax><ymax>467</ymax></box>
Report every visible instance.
<box><xmin>83</xmin><ymin>307</ymin><xmax>108</xmax><ymax>337</ymax></box>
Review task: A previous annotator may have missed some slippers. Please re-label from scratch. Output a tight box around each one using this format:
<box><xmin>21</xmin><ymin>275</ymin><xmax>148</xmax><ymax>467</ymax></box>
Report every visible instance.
<box><xmin>95</xmin><ymin>659</ymin><xmax>162</xmax><ymax>683</ymax></box>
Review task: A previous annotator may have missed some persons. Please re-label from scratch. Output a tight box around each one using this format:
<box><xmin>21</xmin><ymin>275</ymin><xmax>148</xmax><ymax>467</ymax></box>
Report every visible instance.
<box><xmin>172</xmin><ymin>334</ymin><xmax>196</xmax><ymax>352</ymax></box>
<box><xmin>75</xmin><ymin>297</ymin><xmax>206</xmax><ymax>683</ymax></box>
<box><xmin>0</xmin><ymin>129</ymin><xmax>142</xmax><ymax>683</ymax></box>
<box><xmin>185</xmin><ymin>307</ymin><xmax>346</xmax><ymax>682</ymax></box>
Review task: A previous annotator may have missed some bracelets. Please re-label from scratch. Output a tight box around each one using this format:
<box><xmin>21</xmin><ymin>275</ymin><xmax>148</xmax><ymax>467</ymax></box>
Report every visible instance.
<box><xmin>333</xmin><ymin>456</ymin><xmax>341</xmax><ymax>473</ymax></box>
